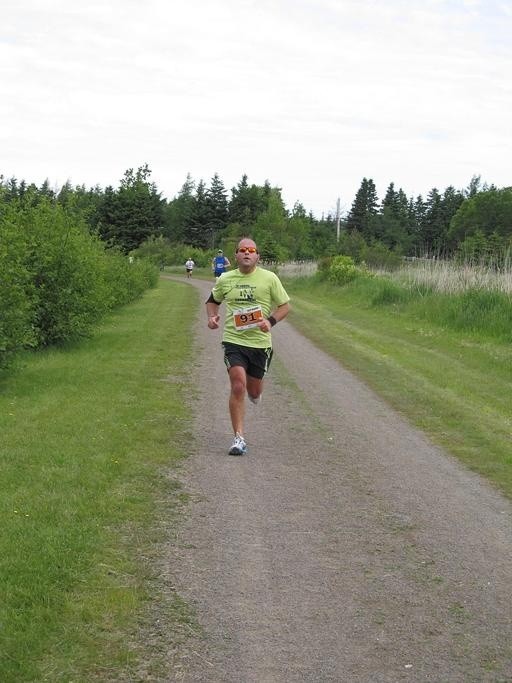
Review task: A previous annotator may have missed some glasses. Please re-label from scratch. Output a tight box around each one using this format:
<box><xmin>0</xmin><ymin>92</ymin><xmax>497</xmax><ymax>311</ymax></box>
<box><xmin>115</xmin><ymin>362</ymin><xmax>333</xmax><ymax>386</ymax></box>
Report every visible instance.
<box><xmin>237</xmin><ymin>246</ymin><xmax>258</xmax><ymax>254</ymax></box>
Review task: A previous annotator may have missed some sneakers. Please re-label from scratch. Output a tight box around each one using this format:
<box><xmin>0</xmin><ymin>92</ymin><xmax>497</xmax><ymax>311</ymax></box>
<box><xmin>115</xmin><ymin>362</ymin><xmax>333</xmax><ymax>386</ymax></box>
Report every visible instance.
<box><xmin>228</xmin><ymin>432</ymin><xmax>247</xmax><ymax>454</ymax></box>
<box><xmin>248</xmin><ymin>395</ymin><xmax>262</xmax><ymax>405</ymax></box>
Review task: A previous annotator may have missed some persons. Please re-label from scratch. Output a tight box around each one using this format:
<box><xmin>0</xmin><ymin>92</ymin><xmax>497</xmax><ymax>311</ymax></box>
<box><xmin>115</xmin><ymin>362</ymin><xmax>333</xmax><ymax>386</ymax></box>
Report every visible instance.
<box><xmin>203</xmin><ymin>236</ymin><xmax>292</xmax><ymax>455</ymax></box>
<box><xmin>185</xmin><ymin>258</ymin><xmax>195</xmax><ymax>278</ymax></box>
<box><xmin>211</xmin><ymin>249</ymin><xmax>231</xmax><ymax>284</ymax></box>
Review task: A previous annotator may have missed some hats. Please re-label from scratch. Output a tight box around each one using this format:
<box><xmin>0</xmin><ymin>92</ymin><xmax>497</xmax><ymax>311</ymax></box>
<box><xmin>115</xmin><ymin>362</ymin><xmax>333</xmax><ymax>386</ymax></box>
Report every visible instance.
<box><xmin>218</xmin><ymin>249</ymin><xmax>222</xmax><ymax>252</ymax></box>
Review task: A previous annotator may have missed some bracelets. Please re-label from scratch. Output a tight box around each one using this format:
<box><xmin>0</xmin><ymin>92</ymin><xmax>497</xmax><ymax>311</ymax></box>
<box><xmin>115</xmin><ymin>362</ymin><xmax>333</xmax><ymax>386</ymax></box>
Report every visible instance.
<box><xmin>268</xmin><ymin>316</ymin><xmax>277</xmax><ymax>327</ymax></box>
